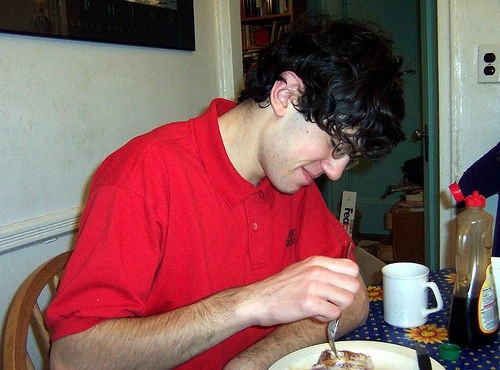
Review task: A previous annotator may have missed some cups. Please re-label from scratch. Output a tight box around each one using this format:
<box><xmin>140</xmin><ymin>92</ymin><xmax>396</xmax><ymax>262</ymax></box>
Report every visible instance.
<box><xmin>382</xmin><ymin>262</ymin><xmax>443</xmax><ymax>328</ymax></box>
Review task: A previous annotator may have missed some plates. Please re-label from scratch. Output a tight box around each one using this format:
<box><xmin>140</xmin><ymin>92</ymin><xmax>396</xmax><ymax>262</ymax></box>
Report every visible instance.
<box><xmin>267</xmin><ymin>340</ymin><xmax>446</xmax><ymax>370</ymax></box>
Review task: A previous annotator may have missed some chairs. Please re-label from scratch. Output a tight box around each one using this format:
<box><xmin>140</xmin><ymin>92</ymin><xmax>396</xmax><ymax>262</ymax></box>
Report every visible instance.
<box><xmin>0</xmin><ymin>250</ymin><xmax>74</xmax><ymax>370</ymax></box>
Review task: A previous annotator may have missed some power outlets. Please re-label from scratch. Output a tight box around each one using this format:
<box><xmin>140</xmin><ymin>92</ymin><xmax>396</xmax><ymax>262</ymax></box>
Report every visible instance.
<box><xmin>477</xmin><ymin>46</ymin><xmax>500</xmax><ymax>84</ymax></box>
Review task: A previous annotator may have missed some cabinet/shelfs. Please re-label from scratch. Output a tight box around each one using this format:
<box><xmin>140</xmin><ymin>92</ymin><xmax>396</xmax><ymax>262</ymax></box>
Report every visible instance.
<box><xmin>239</xmin><ymin>1</ymin><xmax>294</xmax><ymax>85</ymax></box>
<box><xmin>386</xmin><ymin>201</ymin><xmax>425</xmax><ymax>266</ymax></box>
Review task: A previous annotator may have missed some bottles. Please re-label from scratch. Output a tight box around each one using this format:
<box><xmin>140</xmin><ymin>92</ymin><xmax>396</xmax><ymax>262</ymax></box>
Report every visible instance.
<box><xmin>447</xmin><ymin>181</ymin><xmax>499</xmax><ymax>349</ymax></box>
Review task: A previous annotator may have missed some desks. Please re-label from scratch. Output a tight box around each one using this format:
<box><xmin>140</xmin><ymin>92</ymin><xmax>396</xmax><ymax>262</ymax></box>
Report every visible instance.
<box><xmin>334</xmin><ymin>266</ymin><xmax>500</xmax><ymax>370</ymax></box>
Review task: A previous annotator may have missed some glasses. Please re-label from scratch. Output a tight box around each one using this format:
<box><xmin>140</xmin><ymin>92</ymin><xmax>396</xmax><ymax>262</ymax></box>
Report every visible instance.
<box><xmin>324</xmin><ymin>114</ymin><xmax>358</xmax><ymax>170</ymax></box>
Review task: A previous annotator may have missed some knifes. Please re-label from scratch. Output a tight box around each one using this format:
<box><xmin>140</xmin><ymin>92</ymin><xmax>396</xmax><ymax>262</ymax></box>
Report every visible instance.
<box><xmin>417</xmin><ymin>347</ymin><xmax>433</xmax><ymax>370</ymax></box>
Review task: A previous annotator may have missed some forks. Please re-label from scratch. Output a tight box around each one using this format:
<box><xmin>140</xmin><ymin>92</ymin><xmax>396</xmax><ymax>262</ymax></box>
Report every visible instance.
<box><xmin>327</xmin><ymin>240</ymin><xmax>353</xmax><ymax>359</ymax></box>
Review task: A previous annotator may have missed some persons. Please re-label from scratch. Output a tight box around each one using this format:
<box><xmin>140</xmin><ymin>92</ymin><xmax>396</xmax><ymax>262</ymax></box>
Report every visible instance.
<box><xmin>43</xmin><ymin>15</ymin><xmax>405</xmax><ymax>370</ymax></box>
